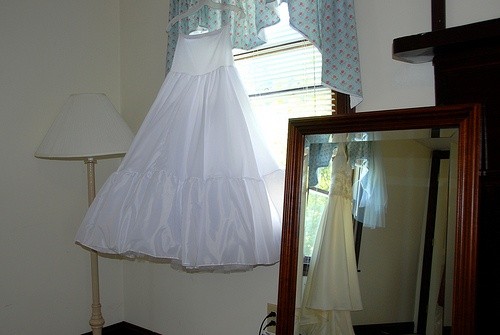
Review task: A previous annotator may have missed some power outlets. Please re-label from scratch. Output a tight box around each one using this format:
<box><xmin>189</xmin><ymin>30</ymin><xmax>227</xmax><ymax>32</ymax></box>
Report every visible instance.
<box><xmin>266</xmin><ymin>303</ymin><xmax>277</xmax><ymax>333</ymax></box>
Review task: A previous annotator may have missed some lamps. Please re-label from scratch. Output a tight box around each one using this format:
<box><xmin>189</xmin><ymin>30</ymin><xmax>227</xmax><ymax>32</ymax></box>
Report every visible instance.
<box><xmin>33</xmin><ymin>93</ymin><xmax>135</xmax><ymax>335</ymax></box>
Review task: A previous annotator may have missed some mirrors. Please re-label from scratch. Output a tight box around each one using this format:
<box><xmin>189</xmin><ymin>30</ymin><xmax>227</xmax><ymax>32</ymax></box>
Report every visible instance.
<box><xmin>278</xmin><ymin>103</ymin><xmax>484</xmax><ymax>335</ymax></box>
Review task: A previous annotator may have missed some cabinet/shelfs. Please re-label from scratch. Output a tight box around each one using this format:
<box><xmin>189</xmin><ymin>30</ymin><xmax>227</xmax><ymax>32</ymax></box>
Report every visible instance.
<box><xmin>390</xmin><ymin>17</ymin><xmax>499</xmax><ymax>335</ymax></box>
